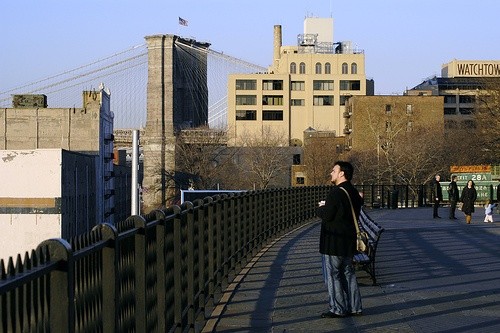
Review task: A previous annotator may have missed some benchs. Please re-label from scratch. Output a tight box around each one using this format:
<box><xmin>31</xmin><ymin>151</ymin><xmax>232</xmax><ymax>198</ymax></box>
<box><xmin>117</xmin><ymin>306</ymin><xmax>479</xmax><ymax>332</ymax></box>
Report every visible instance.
<box><xmin>350</xmin><ymin>206</ymin><xmax>385</xmax><ymax>286</ymax></box>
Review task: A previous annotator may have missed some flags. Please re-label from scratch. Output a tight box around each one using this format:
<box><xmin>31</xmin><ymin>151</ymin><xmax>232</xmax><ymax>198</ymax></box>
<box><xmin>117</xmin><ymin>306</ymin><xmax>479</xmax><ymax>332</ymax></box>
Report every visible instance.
<box><xmin>179</xmin><ymin>16</ymin><xmax>188</xmax><ymax>26</ymax></box>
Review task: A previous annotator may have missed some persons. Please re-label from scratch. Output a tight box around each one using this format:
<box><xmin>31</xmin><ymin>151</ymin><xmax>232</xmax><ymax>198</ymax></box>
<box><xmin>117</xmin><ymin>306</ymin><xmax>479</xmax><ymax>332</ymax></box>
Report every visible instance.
<box><xmin>316</xmin><ymin>161</ymin><xmax>362</xmax><ymax>318</ymax></box>
<box><xmin>447</xmin><ymin>175</ymin><xmax>460</xmax><ymax>220</ymax></box>
<box><xmin>461</xmin><ymin>180</ymin><xmax>477</xmax><ymax>224</ymax></box>
<box><xmin>431</xmin><ymin>174</ymin><xmax>443</xmax><ymax>219</ymax></box>
<box><xmin>483</xmin><ymin>199</ymin><xmax>496</xmax><ymax>223</ymax></box>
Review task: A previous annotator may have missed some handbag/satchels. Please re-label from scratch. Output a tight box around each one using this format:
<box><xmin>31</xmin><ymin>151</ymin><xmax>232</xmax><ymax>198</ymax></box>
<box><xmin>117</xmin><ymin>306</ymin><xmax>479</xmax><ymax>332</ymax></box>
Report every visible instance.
<box><xmin>357</xmin><ymin>232</ymin><xmax>368</xmax><ymax>252</ymax></box>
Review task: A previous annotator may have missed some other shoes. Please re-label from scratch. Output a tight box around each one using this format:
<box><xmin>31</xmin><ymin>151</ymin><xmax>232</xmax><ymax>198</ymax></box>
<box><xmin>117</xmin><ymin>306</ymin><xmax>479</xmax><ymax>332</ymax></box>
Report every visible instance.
<box><xmin>433</xmin><ymin>217</ymin><xmax>441</xmax><ymax>219</ymax></box>
<box><xmin>450</xmin><ymin>217</ymin><xmax>457</xmax><ymax>220</ymax></box>
<box><xmin>321</xmin><ymin>311</ymin><xmax>341</xmax><ymax>317</ymax></box>
<box><xmin>466</xmin><ymin>215</ymin><xmax>471</xmax><ymax>224</ymax></box>
